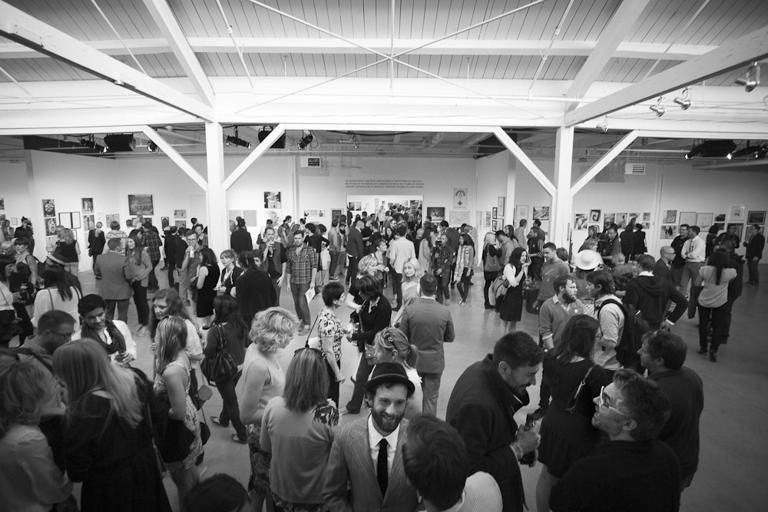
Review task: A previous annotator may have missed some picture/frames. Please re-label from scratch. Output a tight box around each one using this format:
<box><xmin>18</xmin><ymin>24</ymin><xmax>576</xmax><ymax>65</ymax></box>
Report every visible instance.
<box><xmin>82</xmin><ymin>197</ymin><xmax>93</xmax><ymax>211</ymax></box>
<box><xmin>174</xmin><ymin>210</ymin><xmax>187</xmax><ymax>218</ymax></box>
<box><xmin>516</xmin><ymin>204</ymin><xmax>529</xmax><ymax>222</ymax></box>
<box><xmin>663</xmin><ymin>210</ymin><xmax>677</xmax><ymax>223</ymax></box>
<box><xmin>105</xmin><ymin>214</ymin><xmax>120</xmax><ymax>228</ymax></box>
<box><xmin>659</xmin><ymin>225</ymin><xmax>678</xmax><ymax>239</ymax></box>
<box><xmin>604</xmin><ymin>213</ymin><xmax>615</xmax><ymax>228</ymax></box>
<box><xmin>497</xmin><ymin>196</ymin><xmax>504</xmax><ymax>218</ymax></box>
<box><xmin>70</xmin><ymin>212</ymin><xmax>81</xmax><ymax>229</ymax></box>
<box><xmin>485</xmin><ymin>211</ymin><xmax>491</xmax><ymax>229</ymax></box>
<box><xmin>497</xmin><ymin>219</ymin><xmax>503</xmax><ymax>232</ymax></box>
<box><xmin>42</xmin><ymin>198</ymin><xmax>55</xmax><ymax>217</ymax></box>
<box><xmin>128</xmin><ymin>194</ymin><xmax>154</xmax><ymax>215</ymax></box>
<box><xmin>679</xmin><ymin>211</ymin><xmax>698</xmax><ymax>226</ymax></box>
<box><xmin>617</xmin><ymin>213</ymin><xmax>628</xmax><ymax>228</ymax></box>
<box><xmin>747</xmin><ymin>210</ymin><xmax>767</xmax><ymax>225</ymax></box>
<box><xmin>744</xmin><ymin>225</ymin><xmax>764</xmax><ymax>244</ymax></box>
<box><xmin>175</xmin><ymin>220</ymin><xmax>186</xmax><ymax>230</ymax></box>
<box><xmin>229</xmin><ymin>209</ymin><xmax>241</xmax><ymax>225</ymax></box>
<box><xmin>264</xmin><ymin>191</ymin><xmax>282</xmax><ymax>208</ymax></box>
<box><xmin>643</xmin><ymin>213</ymin><xmax>650</xmax><ymax>220</ymax></box>
<box><xmin>728</xmin><ymin>203</ymin><xmax>746</xmax><ymax>220</ymax></box>
<box><xmin>727</xmin><ymin>222</ymin><xmax>743</xmax><ymax>239</ymax></box>
<box><xmin>82</xmin><ymin>214</ymin><xmax>95</xmax><ymax>230</ymax></box>
<box><xmin>318</xmin><ymin>210</ymin><xmax>325</xmax><ymax>217</ymax></box>
<box><xmin>453</xmin><ymin>187</ymin><xmax>468</xmax><ymax>209</ymax></box>
<box><xmin>590</xmin><ymin>209</ymin><xmax>601</xmax><ymax>224</ymax></box>
<box><xmin>533</xmin><ymin>206</ymin><xmax>550</xmax><ymax>221</ymax></box>
<box><xmin>491</xmin><ymin>220</ymin><xmax>496</xmax><ymax>232</ymax></box>
<box><xmin>574</xmin><ymin>213</ymin><xmax>588</xmax><ymax>231</ymax></box>
<box><xmin>243</xmin><ymin>210</ymin><xmax>257</xmax><ymax>226</ymax></box>
<box><xmin>492</xmin><ymin>207</ymin><xmax>497</xmax><ymax>218</ymax></box>
<box><xmin>59</xmin><ymin>211</ymin><xmax>72</xmax><ymax>230</ymax></box>
<box><xmin>45</xmin><ymin>217</ymin><xmax>57</xmax><ymax>235</ymax></box>
<box><xmin>697</xmin><ymin>212</ymin><xmax>713</xmax><ymax>232</ymax></box>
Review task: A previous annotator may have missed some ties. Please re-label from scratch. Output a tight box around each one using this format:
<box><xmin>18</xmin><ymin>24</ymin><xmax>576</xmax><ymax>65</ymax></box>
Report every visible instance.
<box><xmin>378</xmin><ymin>440</ymin><xmax>388</xmax><ymax>497</ymax></box>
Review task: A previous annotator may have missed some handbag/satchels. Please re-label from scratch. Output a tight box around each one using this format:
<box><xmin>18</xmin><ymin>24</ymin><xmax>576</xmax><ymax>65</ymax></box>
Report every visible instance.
<box><xmin>492</xmin><ymin>267</ymin><xmax>519</xmax><ymax>304</ymax></box>
<box><xmin>187</xmin><ymin>365</ymin><xmax>212</xmax><ymax>446</ymax></box>
<box><xmin>88</xmin><ymin>230</ymin><xmax>96</xmax><ymax>256</ymax></box>
<box><xmin>689</xmin><ymin>285</ymin><xmax>705</xmax><ymax>319</ymax></box>
<box><xmin>305</xmin><ymin>307</ymin><xmax>322</xmax><ymax>351</ymax></box>
<box><xmin>484</xmin><ymin>244</ymin><xmax>502</xmax><ymax>272</ymax></box>
<box><xmin>570</xmin><ymin>365</ymin><xmax>598</xmax><ymax>439</ymax></box>
<box><xmin>26</xmin><ymin>253</ymin><xmax>47</xmax><ymax>288</ymax></box>
<box><xmin>199</xmin><ymin>324</ymin><xmax>238</xmax><ymax>386</ymax></box>
<box><xmin>54</xmin><ymin>240</ymin><xmax>62</xmax><ymax>256</ymax></box>
<box><xmin>358</xmin><ymin>299</ymin><xmax>377</xmax><ymax>365</ymax></box>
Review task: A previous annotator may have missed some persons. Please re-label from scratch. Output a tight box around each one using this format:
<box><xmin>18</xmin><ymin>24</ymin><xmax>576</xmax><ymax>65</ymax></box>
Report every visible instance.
<box><xmin>222</xmin><ymin>204</ymin><xmax>475</xmax><ymax>328</ymax></box>
<box><xmin>53</xmin><ymin>339</ymin><xmax>172</xmax><ymax>511</ymax></box>
<box><xmin>399</xmin><ymin>412</ymin><xmax>503</xmax><ymax>512</ymax></box>
<box><xmin>0</xmin><ymin>254</ymin><xmax>23</xmax><ymax>348</ymax></box>
<box><xmin>742</xmin><ymin>224</ymin><xmax>766</xmax><ymax>289</ymax></box>
<box><xmin>718</xmin><ymin>224</ymin><xmax>739</xmax><ymax>252</ymax></box>
<box><xmin>58</xmin><ymin>229</ymin><xmax>81</xmax><ymax>274</ymax></box>
<box><xmin>679</xmin><ymin>226</ymin><xmax>705</xmax><ymax>296</ymax></box>
<box><xmin>602</xmin><ymin>224</ymin><xmax>621</xmax><ymax>259</ymax></box>
<box><xmin>619</xmin><ymin>223</ymin><xmax>634</xmax><ymax>263</ymax></box>
<box><xmin>151</xmin><ymin>316</ymin><xmax>204</xmax><ymax>495</ymax></box>
<box><xmin>585</xmin><ymin>271</ymin><xmax>624</xmax><ymax>372</ymax></box>
<box><xmin>318</xmin><ymin>361</ymin><xmax>417</xmax><ymax>512</ymax></box>
<box><xmin>556</xmin><ymin>247</ymin><xmax>567</xmax><ymax>262</ymax></box>
<box><xmin>634</xmin><ymin>223</ymin><xmax>646</xmax><ymax>256</ymax></box>
<box><xmin>398</xmin><ymin>274</ymin><xmax>456</xmax><ymax>416</ymax></box>
<box><xmin>548</xmin><ymin>369</ymin><xmax>681</xmax><ymax>512</ymax></box>
<box><xmin>623</xmin><ymin>253</ymin><xmax>688</xmax><ymax>332</ymax></box>
<box><xmin>350</xmin><ymin>309</ymin><xmax>374</xmax><ymax>384</ymax></box>
<box><xmin>70</xmin><ymin>294</ymin><xmax>136</xmax><ymax>374</ymax></box>
<box><xmin>536</xmin><ymin>242</ymin><xmax>570</xmax><ymax>347</ymax></box>
<box><xmin>481</xmin><ymin>231</ymin><xmax>502</xmax><ymax>310</ymax></box>
<box><xmin>514</xmin><ymin>219</ymin><xmax>528</xmax><ymax>250</ymax></box>
<box><xmin>587</xmin><ymin>225</ymin><xmax>601</xmax><ymax>239</ymax></box>
<box><xmin>150</xmin><ymin>288</ymin><xmax>205</xmax><ymax>410</ymax></box>
<box><xmin>706</xmin><ymin>224</ymin><xmax>719</xmax><ymax>256</ymax></box>
<box><xmin>527</xmin><ymin>227</ymin><xmax>545</xmax><ymax>280</ymax></box>
<box><xmin>46</xmin><ymin>224</ymin><xmax>64</xmax><ymax>251</ymax></box>
<box><xmin>532</xmin><ymin>274</ymin><xmax>583</xmax><ymax>419</ymax></box>
<box><xmin>535</xmin><ymin>315</ymin><xmax>614</xmax><ymax>512</ymax></box>
<box><xmin>373</xmin><ymin>327</ymin><xmax>422</xmax><ymax>416</ymax></box>
<box><xmin>8</xmin><ymin>237</ymin><xmax>42</xmax><ymax>340</ymax></box>
<box><xmin>653</xmin><ymin>245</ymin><xmax>676</xmax><ymax>281</ymax></box>
<box><xmin>236</xmin><ymin>306</ymin><xmax>301</xmax><ymax>506</ymax></box>
<box><xmin>15</xmin><ymin>217</ymin><xmax>28</xmax><ymax>238</ymax></box>
<box><xmin>612</xmin><ymin>252</ymin><xmax>628</xmax><ymax>275</ymax></box>
<box><xmin>671</xmin><ymin>223</ymin><xmax>690</xmax><ymax>289</ymax></box>
<box><xmin>446</xmin><ymin>330</ymin><xmax>542</xmax><ymax>512</ymax></box>
<box><xmin>342</xmin><ymin>275</ymin><xmax>392</xmax><ymax>415</ymax></box>
<box><xmin>504</xmin><ymin>225</ymin><xmax>520</xmax><ymax>247</ymax></box>
<box><xmin>695</xmin><ymin>249</ymin><xmax>737</xmax><ymax>363</ymax></box>
<box><xmin>722</xmin><ymin>240</ymin><xmax>743</xmax><ymax>343</ymax></box>
<box><xmin>201</xmin><ymin>296</ymin><xmax>247</xmax><ymax>444</ymax></box>
<box><xmin>162</xmin><ymin>213</ymin><xmax>222</xmax><ymax>299</ymax></box>
<box><xmin>28</xmin><ymin>264</ymin><xmax>84</xmax><ymax>343</ymax></box>
<box><xmin>5</xmin><ymin>220</ymin><xmax>14</xmax><ymax>235</ymax></box>
<box><xmin>705</xmin><ymin>244</ymin><xmax>720</xmax><ymax>266</ymax></box>
<box><xmin>572</xmin><ymin>249</ymin><xmax>600</xmax><ymax>303</ymax></box>
<box><xmin>636</xmin><ymin>329</ymin><xmax>705</xmax><ymax>491</ymax></box>
<box><xmin>15</xmin><ymin>310</ymin><xmax>75</xmax><ymax>370</ymax></box>
<box><xmin>317</xmin><ymin>282</ymin><xmax>345</xmax><ymax>409</ymax></box>
<box><xmin>499</xmin><ymin>248</ymin><xmax>532</xmax><ymax>333</ymax></box>
<box><xmin>615</xmin><ymin>255</ymin><xmax>640</xmax><ymax>296</ymax></box>
<box><xmin>496</xmin><ymin>230</ymin><xmax>514</xmax><ymax>269</ymax></box>
<box><xmin>1</xmin><ymin>358</ymin><xmax>76</xmax><ymax>512</ymax></box>
<box><xmin>260</xmin><ymin>346</ymin><xmax>342</xmax><ymax>511</ymax></box>
<box><xmin>183</xmin><ymin>471</ymin><xmax>250</xmax><ymax>512</ymax></box>
<box><xmin>533</xmin><ymin>220</ymin><xmax>545</xmax><ymax>240</ymax></box>
<box><xmin>88</xmin><ymin>214</ymin><xmax>163</xmax><ymax>325</ymax></box>
<box><xmin>584</xmin><ymin>238</ymin><xmax>598</xmax><ymax>250</ymax></box>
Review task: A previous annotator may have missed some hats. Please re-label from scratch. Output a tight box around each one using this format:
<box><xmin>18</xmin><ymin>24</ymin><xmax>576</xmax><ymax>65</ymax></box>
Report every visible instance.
<box><xmin>578</xmin><ymin>251</ymin><xmax>601</xmax><ymax>269</ymax></box>
<box><xmin>364</xmin><ymin>361</ymin><xmax>415</xmax><ymax>399</ymax></box>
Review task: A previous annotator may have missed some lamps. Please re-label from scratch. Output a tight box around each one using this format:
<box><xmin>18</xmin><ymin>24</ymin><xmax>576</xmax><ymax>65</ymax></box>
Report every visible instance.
<box><xmin>297</xmin><ymin>131</ymin><xmax>313</xmax><ymax>149</ymax></box>
<box><xmin>148</xmin><ymin>141</ymin><xmax>158</xmax><ymax>153</ymax></box>
<box><xmin>728</xmin><ymin>140</ymin><xmax>759</xmax><ymax>162</ymax></box>
<box><xmin>684</xmin><ymin>139</ymin><xmax>703</xmax><ymax>161</ymax></box>
<box><xmin>227</xmin><ymin>127</ymin><xmax>250</xmax><ymax>149</ymax></box>
<box><xmin>756</xmin><ymin>139</ymin><xmax>767</xmax><ymax>160</ymax></box>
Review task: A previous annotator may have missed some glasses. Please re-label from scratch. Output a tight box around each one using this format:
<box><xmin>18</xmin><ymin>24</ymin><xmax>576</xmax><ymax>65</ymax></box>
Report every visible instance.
<box><xmin>52</xmin><ymin>329</ymin><xmax>75</xmax><ymax>341</ymax></box>
<box><xmin>600</xmin><ymin>385</ymin><xmax>632</xmax><ymax>420</ymax></box>
<box><xmin>295</xmin><ymin>348</ymin><xmax>325</xmax><ymax>364</ymax></box>
<box><xmin>382</xmin><ymin>329</ymin><xmax>399</xmax><ymax>352</ymax></box>
<box><xmin>9</xmin><ymin>345</ymin><xmax>54</xmax><ymax>374</ymax></box>
<box><xmin>597</xmin><ymin>332</ymin><xmax>602</xmax><ymax>338</ymax></box>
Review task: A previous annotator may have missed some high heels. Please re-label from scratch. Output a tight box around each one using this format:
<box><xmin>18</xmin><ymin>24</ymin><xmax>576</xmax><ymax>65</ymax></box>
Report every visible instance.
<box><xmin>698</xmin><ymin>346</ymin><xmax>706</xmax><ymax>353</ymax></box>
<box><xmin>710</xmin><ymin>348</ymin><xmax>716</xmax><ymax>363</ymax></box>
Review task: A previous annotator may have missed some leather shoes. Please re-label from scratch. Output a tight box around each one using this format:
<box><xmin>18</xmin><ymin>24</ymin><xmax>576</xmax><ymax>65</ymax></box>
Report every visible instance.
<box><xmin>211</xmin><ymin>416</ymin><xmax>222</xmax><ymax>425</ymax></box>
<box><xmin>230</xmin><ymin>434</ymin><xmax>245</xmax><ymax>444</ymax></box>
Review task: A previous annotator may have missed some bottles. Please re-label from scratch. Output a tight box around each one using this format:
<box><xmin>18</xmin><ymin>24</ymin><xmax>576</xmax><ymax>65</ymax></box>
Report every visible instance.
<box><xmin>353</xmin><ymin>322</ymin><xmax>359</xmax><ymax>334</ymax></box>
<box><xmin>521</xmin><ymin>413</ymin><xmax>534</xmax><ymax>464</ymax></box>
<box><xmin>189</xmin><ymin>241</ymin><xmax>194</xmax><ymax>258</ymax></box>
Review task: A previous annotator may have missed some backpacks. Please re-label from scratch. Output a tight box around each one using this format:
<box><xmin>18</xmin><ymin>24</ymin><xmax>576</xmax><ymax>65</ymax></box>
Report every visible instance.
<box><xmin>598</xmin><ymin>299</ymin><xmax>652</xmax><ymax>375</ymax></box>
